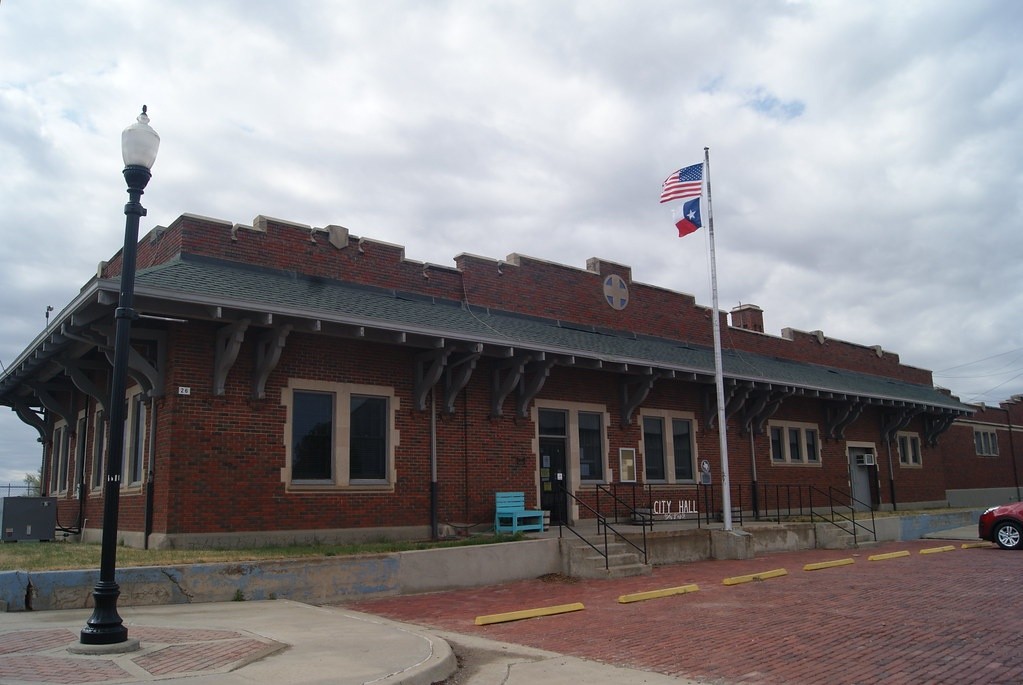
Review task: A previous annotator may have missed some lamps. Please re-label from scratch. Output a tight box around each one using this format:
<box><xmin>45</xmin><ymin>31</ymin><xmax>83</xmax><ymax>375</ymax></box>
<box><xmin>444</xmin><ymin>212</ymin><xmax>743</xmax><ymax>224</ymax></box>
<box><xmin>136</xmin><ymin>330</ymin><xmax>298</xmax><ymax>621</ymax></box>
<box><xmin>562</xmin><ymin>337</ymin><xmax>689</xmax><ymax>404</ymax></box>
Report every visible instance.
<box><xmin>46</xmin><ymin>305</ymin><xmax>54</xmax><ymax>329</ymax></box>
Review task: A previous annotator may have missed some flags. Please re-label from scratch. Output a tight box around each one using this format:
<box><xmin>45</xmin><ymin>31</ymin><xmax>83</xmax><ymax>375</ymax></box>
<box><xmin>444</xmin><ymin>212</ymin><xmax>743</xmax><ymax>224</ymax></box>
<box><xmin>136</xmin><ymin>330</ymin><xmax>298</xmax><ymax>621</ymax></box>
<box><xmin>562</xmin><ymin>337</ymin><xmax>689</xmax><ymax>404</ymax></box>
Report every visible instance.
<box><xmin>660</xmin><ymin>164</ymin><xmax>704</xmax><ymax>203</ymax></box>
<box><xmin>674</xmin><ymin>197</ymin><xmax>702</xmax><ymax>237</ymax></box>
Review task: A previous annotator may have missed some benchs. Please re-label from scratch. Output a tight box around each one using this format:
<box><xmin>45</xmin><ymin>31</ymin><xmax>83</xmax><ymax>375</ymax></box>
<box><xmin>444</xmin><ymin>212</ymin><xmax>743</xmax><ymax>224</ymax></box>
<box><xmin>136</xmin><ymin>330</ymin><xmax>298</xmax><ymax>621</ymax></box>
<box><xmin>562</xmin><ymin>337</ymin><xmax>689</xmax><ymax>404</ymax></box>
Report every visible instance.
<box><xmin>496</xmin><ymin>493</ymin><xmax>545</xmax><ymax>533</ymax></box>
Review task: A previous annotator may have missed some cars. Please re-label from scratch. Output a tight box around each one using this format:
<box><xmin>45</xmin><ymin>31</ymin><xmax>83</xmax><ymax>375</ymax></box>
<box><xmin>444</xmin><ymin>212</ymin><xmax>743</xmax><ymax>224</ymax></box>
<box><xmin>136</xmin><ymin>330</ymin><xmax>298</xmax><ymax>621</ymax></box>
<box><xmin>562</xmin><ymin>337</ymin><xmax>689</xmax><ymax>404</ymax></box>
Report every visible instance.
<box><xmin>979</xmin><ymin>500</ymin><xmax>1023</xmax><ymax>550</ymax></box>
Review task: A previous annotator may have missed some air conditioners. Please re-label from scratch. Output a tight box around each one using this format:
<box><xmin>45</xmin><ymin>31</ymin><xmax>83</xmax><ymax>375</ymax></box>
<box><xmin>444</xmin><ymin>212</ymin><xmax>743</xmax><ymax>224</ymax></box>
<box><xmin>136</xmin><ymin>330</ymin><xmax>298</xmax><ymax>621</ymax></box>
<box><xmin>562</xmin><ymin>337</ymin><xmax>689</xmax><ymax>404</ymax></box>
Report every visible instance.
<box><xmin>858</xmin><ymin>454</ymin><xmax>875</xmax><ymax>465</ymax></box>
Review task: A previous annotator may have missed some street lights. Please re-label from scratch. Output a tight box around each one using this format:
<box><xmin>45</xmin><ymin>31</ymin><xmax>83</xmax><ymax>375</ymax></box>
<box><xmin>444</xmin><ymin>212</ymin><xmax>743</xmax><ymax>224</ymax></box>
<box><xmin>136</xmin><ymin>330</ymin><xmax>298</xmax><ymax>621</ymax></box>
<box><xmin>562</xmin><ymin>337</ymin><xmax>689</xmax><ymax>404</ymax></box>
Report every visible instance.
<box><xmin>79</xmin><ymin>101</ymin><xmax>161</xmax><ymax>646</ymax></box>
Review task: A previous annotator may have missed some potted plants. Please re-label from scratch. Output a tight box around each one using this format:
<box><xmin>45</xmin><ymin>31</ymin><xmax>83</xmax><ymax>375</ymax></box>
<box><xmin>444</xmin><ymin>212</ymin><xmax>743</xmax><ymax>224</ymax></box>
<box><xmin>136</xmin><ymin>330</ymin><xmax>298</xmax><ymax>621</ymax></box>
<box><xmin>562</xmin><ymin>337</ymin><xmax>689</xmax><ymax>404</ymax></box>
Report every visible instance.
<box><xmin>718</xmin><ymin>504</ymin><xmax>743</xmax><ymax>525</ymax></box>
<box><xmin>631</xmin><ymin>500</ymin><xmax>656</xmax><ymax>525</ymax></box>
